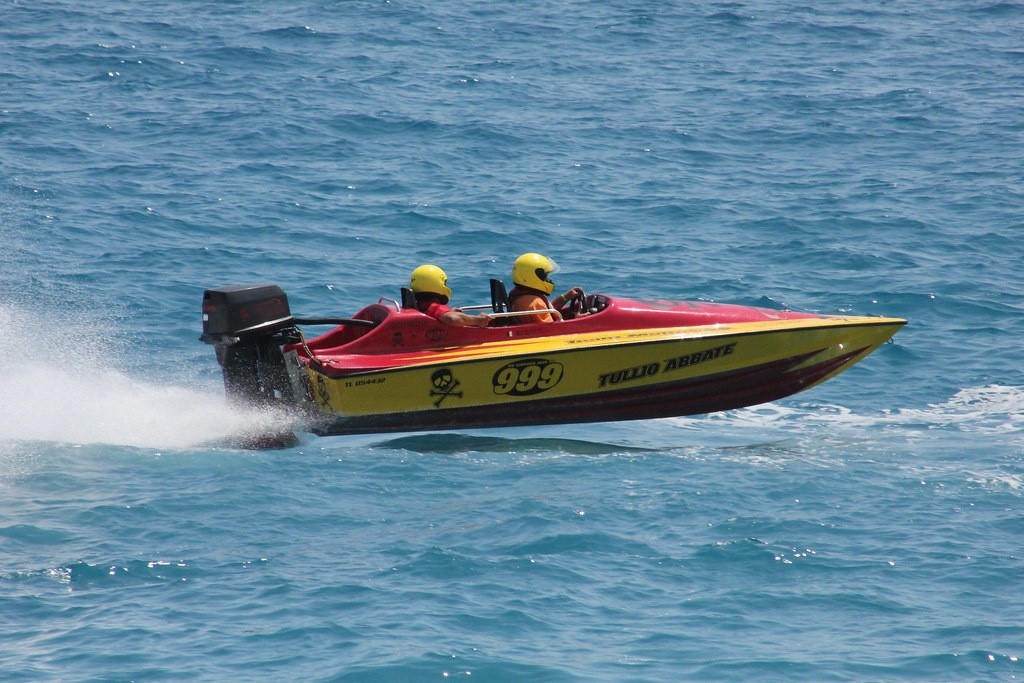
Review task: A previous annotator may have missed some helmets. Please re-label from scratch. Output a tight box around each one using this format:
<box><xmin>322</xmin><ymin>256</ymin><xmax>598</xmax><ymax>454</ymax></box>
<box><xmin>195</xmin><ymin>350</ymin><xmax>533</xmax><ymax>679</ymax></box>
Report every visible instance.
<box><xmin>512</xmin><ymin>254</ymin><xmax>559</xmax><ymax>295</ymax></box>
<box><xmin>408</xmin><ymin>265</ymin><xmax>453</xmax><ymax>306</ymax></box>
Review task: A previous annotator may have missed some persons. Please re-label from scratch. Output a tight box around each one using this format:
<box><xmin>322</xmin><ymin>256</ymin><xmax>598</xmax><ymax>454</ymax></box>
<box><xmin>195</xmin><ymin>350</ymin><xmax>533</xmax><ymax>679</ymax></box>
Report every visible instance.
<box><xmin>408</xmin><ymin>265</ymin><xmax>495</xmax><ymax>328</ymax></box>
<box><xmin>508</xmin><ymin>253</ymin><xmax>581</xmax><ymax>328</ymax></box>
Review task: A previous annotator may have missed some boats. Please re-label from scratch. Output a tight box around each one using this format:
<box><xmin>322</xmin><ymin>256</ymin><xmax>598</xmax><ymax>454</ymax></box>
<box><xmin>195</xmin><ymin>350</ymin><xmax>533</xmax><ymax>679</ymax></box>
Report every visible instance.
<box><xmin>199</xmin><ymin>285</ymin><xmax>910</xmax><ymax>437</ymax></box>
